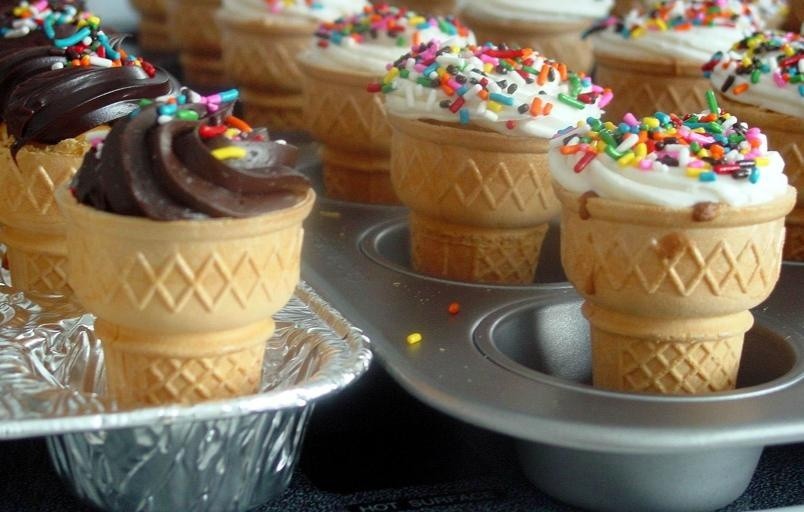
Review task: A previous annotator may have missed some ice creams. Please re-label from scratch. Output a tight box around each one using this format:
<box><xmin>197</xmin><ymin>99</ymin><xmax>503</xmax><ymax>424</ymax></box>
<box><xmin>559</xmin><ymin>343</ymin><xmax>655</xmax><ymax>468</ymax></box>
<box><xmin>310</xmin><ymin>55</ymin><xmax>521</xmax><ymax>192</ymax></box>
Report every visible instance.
<box><xmin>1</xmin><ymin>0</ymin><xmax>804</xmax><ymax>413</ymax></box>
